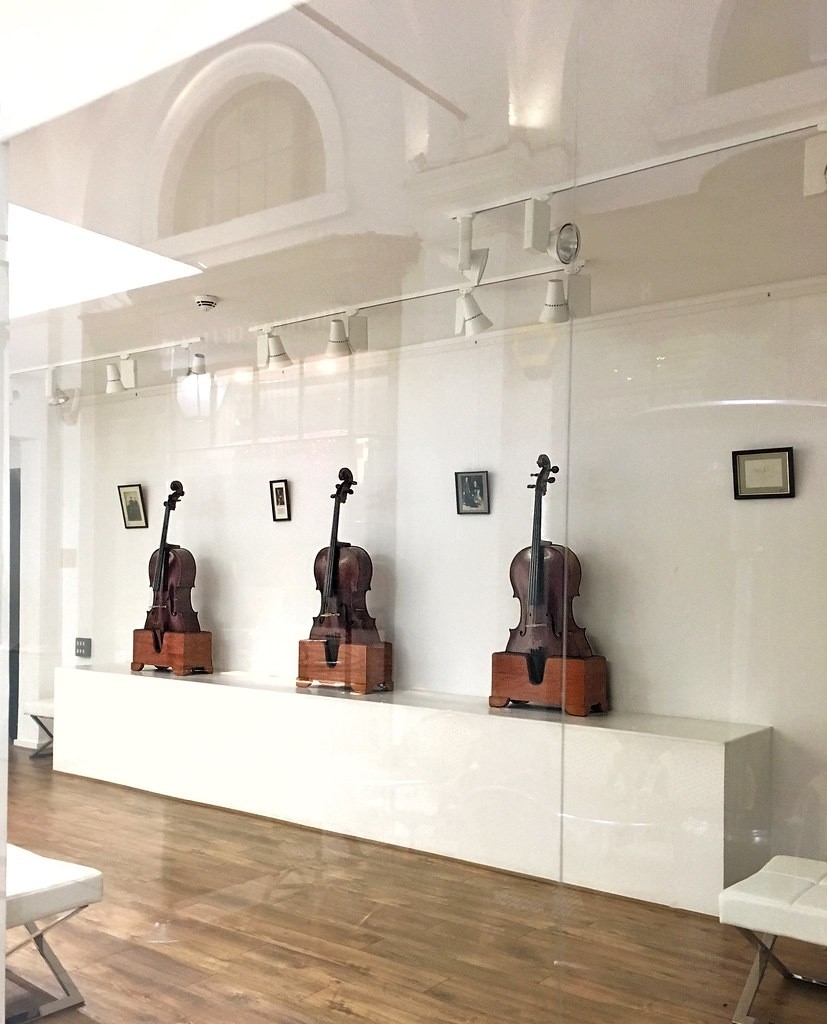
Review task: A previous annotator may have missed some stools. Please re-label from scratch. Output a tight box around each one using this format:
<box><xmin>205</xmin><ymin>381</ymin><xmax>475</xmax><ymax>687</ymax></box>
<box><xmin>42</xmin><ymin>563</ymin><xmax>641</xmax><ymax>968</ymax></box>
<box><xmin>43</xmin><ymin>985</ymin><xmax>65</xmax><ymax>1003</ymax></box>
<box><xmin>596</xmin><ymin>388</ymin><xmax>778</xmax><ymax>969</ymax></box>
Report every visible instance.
<box><xmin>23</xmin><ymin>697</ymin><xmax>54</xmax><ymax>757</ymax></box>
<box><xmin>719</xmin><ymin>854</ymin><xmax>827</xmax><ymax>1024</ymax></box>
<box><xmin>4</xmin><ymin>843</ymin><xmax>104</xmax><ymax>1024</ymax></box>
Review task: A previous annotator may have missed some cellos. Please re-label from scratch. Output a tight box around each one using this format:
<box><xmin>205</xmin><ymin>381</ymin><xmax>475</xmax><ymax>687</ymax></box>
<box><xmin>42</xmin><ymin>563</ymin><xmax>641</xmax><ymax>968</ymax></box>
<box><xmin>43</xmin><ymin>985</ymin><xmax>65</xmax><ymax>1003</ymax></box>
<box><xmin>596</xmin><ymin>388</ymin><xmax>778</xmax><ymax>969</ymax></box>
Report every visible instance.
<box><xmin>308</xmin><ymin>468</ymin><xmax>382</xmax><ymax>665</ymax></box>
<box><xmin>141</xmin><ymin>481</ymin><xmax>200</xmax><ymax>652</ymax></box>
<box><xmin>504</xmin><ymin>453</ymin><xmax>594</xmax><ymax>684</ymax></box>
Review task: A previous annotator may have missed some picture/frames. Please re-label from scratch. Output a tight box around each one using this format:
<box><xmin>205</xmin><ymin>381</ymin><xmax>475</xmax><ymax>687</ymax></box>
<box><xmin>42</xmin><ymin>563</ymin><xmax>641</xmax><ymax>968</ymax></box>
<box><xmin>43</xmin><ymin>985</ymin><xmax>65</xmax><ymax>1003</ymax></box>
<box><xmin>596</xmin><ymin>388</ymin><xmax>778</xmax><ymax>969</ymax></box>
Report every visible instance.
<box><xmin>269</xmin><ymin>479</ymin><xmax>291</xmax><ymax>521</ymax></box>
<box><xmin>454</xmin><ymin>471</ymin><xmax>490</xmax><ymax>514</ymax></box>
<box><xmin>118</xmin><ymin>484</ymin><xmax>148</xmax><ymax>529</ymax></box>
<box><xmin>732</xmin><ymin>447</ymin><xmax>795</xmax><ymax>500</ymax></box>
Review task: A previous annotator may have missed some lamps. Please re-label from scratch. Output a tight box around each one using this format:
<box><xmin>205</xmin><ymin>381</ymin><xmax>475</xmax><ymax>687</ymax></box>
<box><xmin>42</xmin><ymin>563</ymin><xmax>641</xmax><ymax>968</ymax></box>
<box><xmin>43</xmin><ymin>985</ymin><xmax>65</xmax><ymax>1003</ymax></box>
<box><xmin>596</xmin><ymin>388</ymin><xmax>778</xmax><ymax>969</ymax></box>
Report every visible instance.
<box><xmin>40</xmin><ymin>367</ymin><xmax>69</xmax><ymax>405</ymax></box>
<box><xmin>259</xmin><ymin>330</ymin><xmax>294</xmax><ymax>369</ymax></box>
<box><xmin>521</xmin><ymin>194</ymin><xmax>582</xmax><ymax>265</ymax></box>
<box><xmin>180</xmin><ymin>344</ymin><xmax>206</xmax><ymax>376</ymax></box>
<box><xmin>460</xmin><ymin>289</ymin><xmax>494</xmax><ymax>337</ymax></box>
<box><xmin>539</xmin><ymin>280</ymin><xmax>570</xmax><ymax>324</ymax></box>
<box><xmin>325</xmin><ymin>320</ymin><xmax>352</xmax><ymax>358</ymax></box>
<box><xmin>439</xmin><ymin>214</ymin><xmax>489</xmax><ymax>286</ymax></box>
<box><xmin>106</xmin><ymin>365</ymin><xmax>126</xmax><ymax>393</ymax></box>
